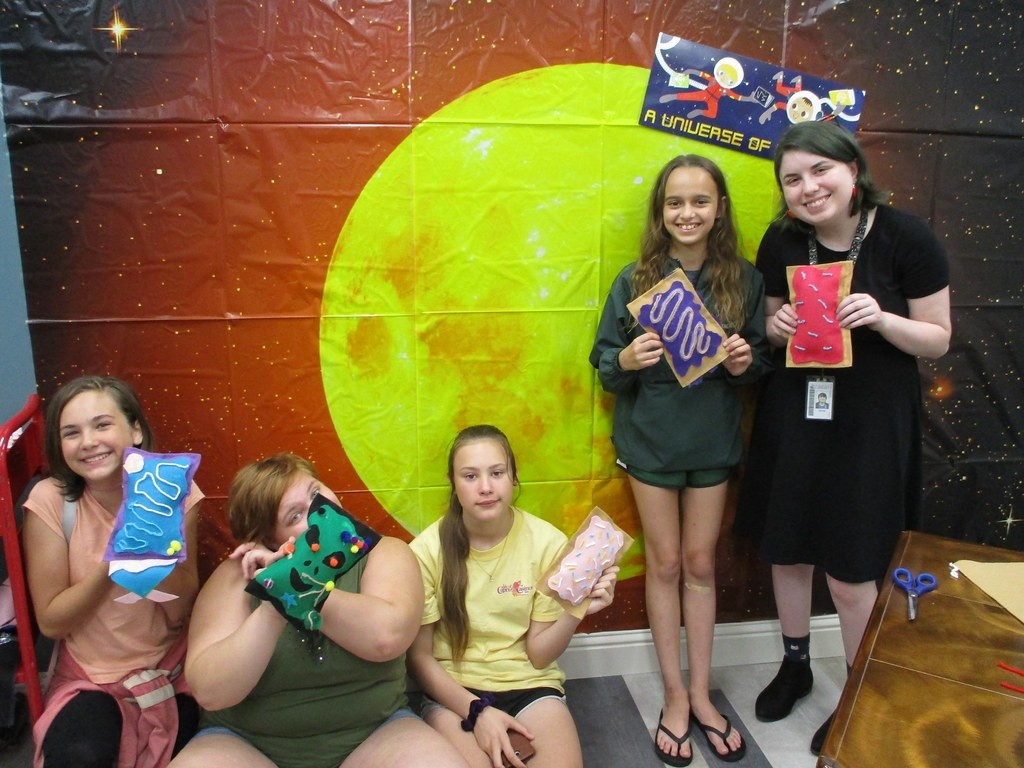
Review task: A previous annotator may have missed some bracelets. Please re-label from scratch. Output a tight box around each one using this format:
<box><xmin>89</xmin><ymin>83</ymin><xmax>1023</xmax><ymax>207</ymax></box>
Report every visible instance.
<box><xmin>461</xmin><ymin>692</ymin><xmax>495</xmax><ymax>733</ymax></box>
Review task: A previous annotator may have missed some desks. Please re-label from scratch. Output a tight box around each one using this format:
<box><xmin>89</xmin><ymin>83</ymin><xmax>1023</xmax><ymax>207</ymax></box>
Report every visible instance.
<box><xmin>815</xmin><ymin>528</ymin><xmax>1024</xmax><ymax>768</ymax></box>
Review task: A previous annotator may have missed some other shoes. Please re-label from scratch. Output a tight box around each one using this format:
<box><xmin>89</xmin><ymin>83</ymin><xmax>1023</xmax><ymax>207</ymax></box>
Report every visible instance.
<box><xmin>811</xmin><ymin>710</ymin><xmax>836</xmax><ymax>755</ymax></box>
<box><xmin>755</xmin><ymin>655</ymin><xmax>813</xmax><ymax>722</ymax></box>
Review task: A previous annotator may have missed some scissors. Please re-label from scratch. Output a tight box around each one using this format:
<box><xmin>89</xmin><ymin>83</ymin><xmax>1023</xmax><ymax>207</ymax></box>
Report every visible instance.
<box><xmin>893</xmin><ymin>568</ymin><xmax>937</xmax><ymax>620</ymax></box>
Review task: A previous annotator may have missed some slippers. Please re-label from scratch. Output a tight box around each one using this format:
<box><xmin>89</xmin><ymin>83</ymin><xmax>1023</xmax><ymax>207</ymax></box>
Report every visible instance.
<box><xmin>690</xmin><ymin>710</ymin><xmax>746</xmax><ymax>762</ymax></box>
<box><xmin>655</xmin><ymin>709</ymin><xmax>693</xmax><ymax>767</ymax></box>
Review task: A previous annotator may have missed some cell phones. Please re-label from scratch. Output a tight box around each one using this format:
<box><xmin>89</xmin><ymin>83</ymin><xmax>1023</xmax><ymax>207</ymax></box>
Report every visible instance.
<box><xmin>500</xmin><ymin>728</ymin><xmax>536</xmax><ymax>768</ymax></box>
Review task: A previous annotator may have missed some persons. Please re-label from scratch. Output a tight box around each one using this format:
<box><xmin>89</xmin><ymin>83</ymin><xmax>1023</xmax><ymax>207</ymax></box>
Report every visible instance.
<box><xmin>164</xmin><ymin>451</ymin><xmax>470</xmax><ymax>768</ymax></box>
<box><xmin>589</xmin><ymin>154</ymin><xmax>772</xmax><ymax>767</ymax></box>
<box><xmin>816</xmin><ymin>393</ymin><xmax>829</xmax><ymax>409</ymax></box>
<box><xmin>730</xmin><ymin>120</ymin><xmax>952</xmax><ymax>756</ymax></box>
<box><xmin>408</xmin><ymin>424</ymin><xmax>620</xmax><ymax>768</ymax></box>
<box><xmin>20</xmin><ymin>375</ymin><xmax>205</xmax><ymax>768</ymax></box>
